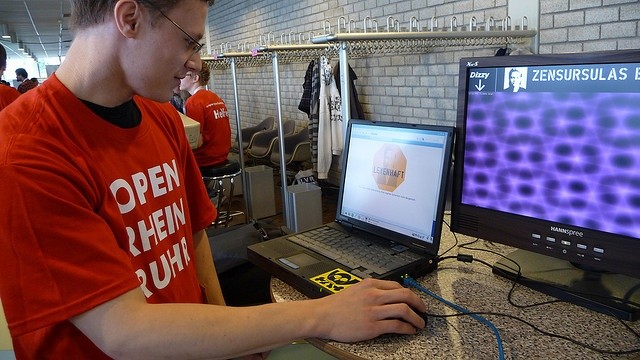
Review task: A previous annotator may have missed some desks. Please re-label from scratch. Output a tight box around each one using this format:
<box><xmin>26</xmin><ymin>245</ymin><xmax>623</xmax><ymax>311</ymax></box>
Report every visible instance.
<box><xmin>270</xmin><ymin>211</ymin><xmax>639</xmax><ymax>360</ymax></box>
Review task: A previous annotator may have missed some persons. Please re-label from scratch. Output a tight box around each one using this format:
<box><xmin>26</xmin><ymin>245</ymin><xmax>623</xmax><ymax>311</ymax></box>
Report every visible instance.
<box><xmin>178</xmin><ymin>59</ymin><xmax>232</xmax><ymax>172</ymax></box>
<box><xmin>1</xmin><ymin>44</ymin><xmax>22</xmax><ymax>112</ymax></box>
<box><xmin>375</xmin><ymin>148</ymin><xmax>399</xmax><ymax>188</ymax></box>
<box><xmin>1</xmin><ymin>0</ymin><xmax>427</xmax><ymax>359</ymax></box>
<box><xmin>15</xmin><ymin>68</ymin><xmax>35</xmax><ymax>93</ymax></box>
<box><xmin>30</xmin><ymin>78</ymin><xmax>39</xmax><ymax>86</ymax></box>
<box><xmin>504</xmin><ymin>68</ymin><xmax>527</xmax><ymax>92</ymax></box>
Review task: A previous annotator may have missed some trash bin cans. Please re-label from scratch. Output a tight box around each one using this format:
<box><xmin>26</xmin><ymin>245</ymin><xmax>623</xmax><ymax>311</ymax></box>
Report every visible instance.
<box><xmin>287</xmin><ymin>183</ymin><xmax>323</xmax><ymax>233</ymax></box>
<box><xmin>245</xmin><ymin>165</ymin><xmax>276</xmax><ymax>220</ymax></box>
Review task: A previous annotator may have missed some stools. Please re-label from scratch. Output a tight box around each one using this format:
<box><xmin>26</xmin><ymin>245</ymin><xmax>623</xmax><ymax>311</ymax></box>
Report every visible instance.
<box><xmin>197</xmin><ymin>158</ymin><xmax>244</xmax><ymax>228</ymax></box>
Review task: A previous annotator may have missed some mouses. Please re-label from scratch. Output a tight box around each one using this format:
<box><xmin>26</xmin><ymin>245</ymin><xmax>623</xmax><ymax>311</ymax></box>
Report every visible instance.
<box><xmin>376</xmin><ymin>305</ymin><xmax>428</xmax><ymax>338</ymax></box>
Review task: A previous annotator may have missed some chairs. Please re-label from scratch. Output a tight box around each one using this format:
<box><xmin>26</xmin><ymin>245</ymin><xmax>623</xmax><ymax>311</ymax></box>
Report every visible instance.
<box><xmin>270</xmin><ymin>127</ymin><xmax>314</xmax><ymax>184</ymax></box>
<box><xmin>232</xmin><ymin>117</ymin><xmax>275</xmax><ymax>152</ymax></box>
<box><xmin>247</xmin><ymin>120</ymin><xmax>296</xmax><ymax>166</ymax></box>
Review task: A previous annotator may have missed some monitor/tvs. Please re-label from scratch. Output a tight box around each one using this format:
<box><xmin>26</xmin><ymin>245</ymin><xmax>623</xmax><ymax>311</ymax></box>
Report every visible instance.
<box><xmin>449</xmin><ymin>49</ymin><xmax>640</xmax><ymax>321</ymax></box>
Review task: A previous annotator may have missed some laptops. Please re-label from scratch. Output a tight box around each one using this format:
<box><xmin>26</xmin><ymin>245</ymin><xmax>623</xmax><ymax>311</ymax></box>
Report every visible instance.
<box><xmin>246</xmin><ymin>120</ymin><xmax>456</xmax><ymax>300</ymax></box>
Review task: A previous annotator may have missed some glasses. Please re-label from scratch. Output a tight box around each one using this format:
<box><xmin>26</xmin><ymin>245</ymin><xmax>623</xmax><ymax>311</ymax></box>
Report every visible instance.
<box><xmin>149</xmin><ymin>3</ymin><xmax>205</xmax><ymax>54</ymax></box>
